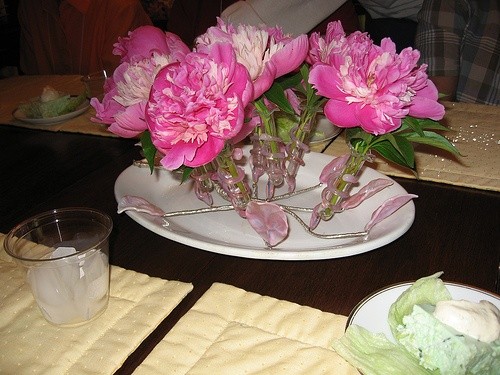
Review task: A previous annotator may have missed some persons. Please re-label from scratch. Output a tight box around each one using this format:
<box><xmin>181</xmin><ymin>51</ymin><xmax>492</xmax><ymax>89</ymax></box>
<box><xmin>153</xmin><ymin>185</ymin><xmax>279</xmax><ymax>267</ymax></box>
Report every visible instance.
<box><xmin>220</xmin><ymin>0</ymin><xmax>424</xmax><ymax>37</ymax></box>
<box><xmin>16</xmin><ymin>0</ymin><xmax>154</xmax><ymax>76</ymax></box>
<box><xmin>415</xmin><ymin>0</ymin><xmax>500</xmax><ymax>105</ymax></box>
<box><xmin>167</xmin><ymin>0</ymin><xmax>239</xmax><ymax>51</ymax></box>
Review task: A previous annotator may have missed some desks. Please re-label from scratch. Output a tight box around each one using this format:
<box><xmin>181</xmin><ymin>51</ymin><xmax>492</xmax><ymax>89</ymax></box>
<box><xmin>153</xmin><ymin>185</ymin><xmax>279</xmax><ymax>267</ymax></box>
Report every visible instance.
<box><xmin>0</xmin><ymin>74</ymin><xmax>500</xmax><ymax>375</ymax></box>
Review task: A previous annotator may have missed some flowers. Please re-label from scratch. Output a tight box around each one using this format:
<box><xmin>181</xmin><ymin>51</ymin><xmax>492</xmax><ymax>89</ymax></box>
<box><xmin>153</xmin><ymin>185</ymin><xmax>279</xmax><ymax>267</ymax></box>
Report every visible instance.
<box><xmin>90</xmin><ymin>16</ymin><xmax>468</xmax><ymax>247</ymax></box>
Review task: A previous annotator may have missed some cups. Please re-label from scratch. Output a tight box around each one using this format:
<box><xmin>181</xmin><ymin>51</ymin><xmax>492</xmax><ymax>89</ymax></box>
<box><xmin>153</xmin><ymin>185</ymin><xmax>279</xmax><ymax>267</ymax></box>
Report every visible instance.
<box><xmin>2</xmin><ymin>206</ymin><xmax>115</xmax><ymax>328</ymax></box>
<box><xmin>80</xmin><ymin>64</ymin><xmax>118</xmax><ymax>103</ymax></box>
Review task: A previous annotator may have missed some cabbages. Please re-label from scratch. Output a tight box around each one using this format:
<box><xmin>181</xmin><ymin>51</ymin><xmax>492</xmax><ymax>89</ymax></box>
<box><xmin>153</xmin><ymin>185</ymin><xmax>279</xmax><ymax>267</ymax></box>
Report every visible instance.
<box><xmin>334</xmin><ymin>271</ymin><xmax>500</xmax><ymax>375</ymax></box>
<box><xmin>17</xmin><ymin>89</ymin><xmax>88</xmax><ymax>119</ymax></box>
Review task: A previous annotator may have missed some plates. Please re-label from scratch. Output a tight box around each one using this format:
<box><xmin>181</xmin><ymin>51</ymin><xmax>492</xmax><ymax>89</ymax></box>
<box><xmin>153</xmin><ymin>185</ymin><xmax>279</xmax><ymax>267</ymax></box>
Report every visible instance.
<box><xmin>308</xmin><ymin>114</ymin><xmax>342</xmax><ymax>146</ymax></box>
<box><xmin>345</xmin><ymin>279</ymin><xmax>500</xmax><ymax>374</ymax></box>
<box><xmin>13</xmin><ymin>94</ymin><xmax>91</xmax><ymax>126</ymax></box>
<box><xmin>115</xmin><ymin>149</ymin><xmax>415</xmax><ymax>260</ymax></box>
